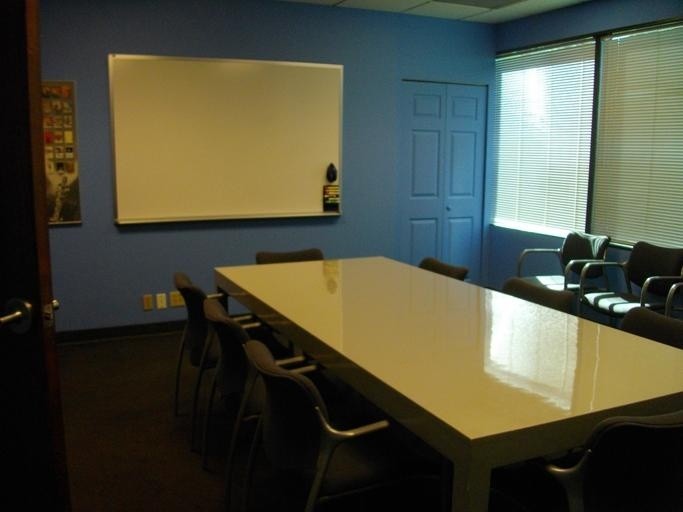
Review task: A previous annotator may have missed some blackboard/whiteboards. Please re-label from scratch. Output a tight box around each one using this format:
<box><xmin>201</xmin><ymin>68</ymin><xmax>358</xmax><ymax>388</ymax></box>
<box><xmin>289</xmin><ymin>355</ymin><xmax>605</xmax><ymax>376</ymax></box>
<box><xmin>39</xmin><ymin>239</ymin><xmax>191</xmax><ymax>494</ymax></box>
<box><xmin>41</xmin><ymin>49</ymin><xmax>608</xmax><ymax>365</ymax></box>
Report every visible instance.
<box><xmin>107</xmin><ymin>52</ymin><xmax>345</xmax><ymax>227</ymax></box>
